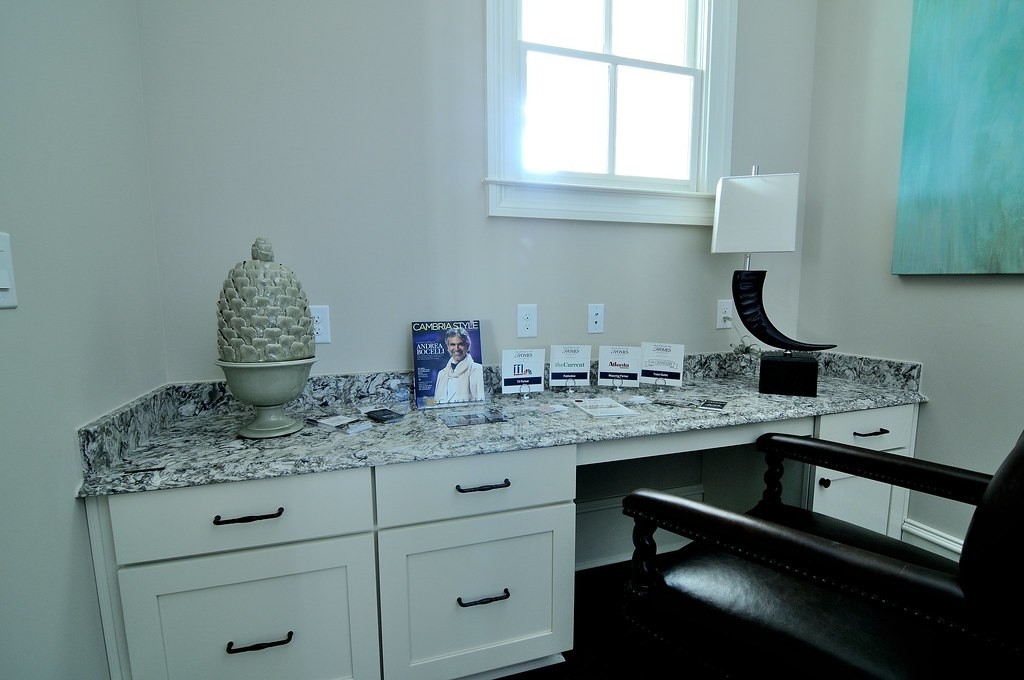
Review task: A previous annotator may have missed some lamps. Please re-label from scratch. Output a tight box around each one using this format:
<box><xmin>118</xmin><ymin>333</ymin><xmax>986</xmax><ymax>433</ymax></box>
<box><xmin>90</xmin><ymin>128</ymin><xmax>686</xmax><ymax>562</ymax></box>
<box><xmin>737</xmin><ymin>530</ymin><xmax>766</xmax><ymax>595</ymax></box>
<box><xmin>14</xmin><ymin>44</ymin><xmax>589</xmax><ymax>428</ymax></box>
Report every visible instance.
<box><xmin>709</xmin><ymin>164</ymin><xmax>839</xmax><ymax>398</ymax></box>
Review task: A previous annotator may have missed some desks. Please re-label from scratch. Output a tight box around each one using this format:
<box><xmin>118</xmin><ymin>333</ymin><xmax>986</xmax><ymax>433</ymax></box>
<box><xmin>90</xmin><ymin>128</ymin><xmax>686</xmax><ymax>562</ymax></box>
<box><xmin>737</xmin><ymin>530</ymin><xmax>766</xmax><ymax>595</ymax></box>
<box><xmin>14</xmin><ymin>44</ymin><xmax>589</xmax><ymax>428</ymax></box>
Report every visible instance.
<box><xmin>73</xmin><ymin>349</ymin><xmax>930</xmax><ymax>680</ymax></box>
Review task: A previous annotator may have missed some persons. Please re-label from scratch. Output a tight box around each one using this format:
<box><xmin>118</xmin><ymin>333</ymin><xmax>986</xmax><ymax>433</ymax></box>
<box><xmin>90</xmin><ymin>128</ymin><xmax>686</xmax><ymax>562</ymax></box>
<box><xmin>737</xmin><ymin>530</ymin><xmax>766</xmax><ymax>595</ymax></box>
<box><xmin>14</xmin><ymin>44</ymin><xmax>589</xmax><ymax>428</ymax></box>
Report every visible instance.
<box><xmin>433</xmin><ymin>327</ymin><xmax>484</xmax><ymax>404</ymax></box>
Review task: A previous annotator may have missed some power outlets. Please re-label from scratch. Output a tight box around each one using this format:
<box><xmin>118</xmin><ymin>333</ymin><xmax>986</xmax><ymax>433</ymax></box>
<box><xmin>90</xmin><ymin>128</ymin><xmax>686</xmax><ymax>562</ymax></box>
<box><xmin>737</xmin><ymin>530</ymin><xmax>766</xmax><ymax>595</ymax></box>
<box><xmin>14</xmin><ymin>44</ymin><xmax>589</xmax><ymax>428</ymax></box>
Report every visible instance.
<box><xmin>716</xmin><ymin>300</ymin><xmax>733</xmax><ymax>329</ymax></box>
<box><xmin>308</xmin><ymin>305</ymin><xmax>331</xmax><ymax>344</ymax></box>
<box><xmin>587</xmin><ymin>304</ymin><xmax>605</xmax><ymax>334</ymax></box>
<box><xmin>517</xmin><ymin>304</ymin><xmax>538</xmax><ymax>338</ymax></box>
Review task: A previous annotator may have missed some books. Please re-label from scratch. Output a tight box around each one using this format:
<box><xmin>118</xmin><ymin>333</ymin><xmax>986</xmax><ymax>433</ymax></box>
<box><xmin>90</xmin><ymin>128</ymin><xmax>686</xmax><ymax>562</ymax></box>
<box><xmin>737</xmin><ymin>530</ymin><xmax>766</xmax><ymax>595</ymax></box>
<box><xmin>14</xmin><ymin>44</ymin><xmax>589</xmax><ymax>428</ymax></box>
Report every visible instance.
<box><xmin>411</xmin><ymin>320</ymin><xmax>485</xmax><ymax>410</ymax></box>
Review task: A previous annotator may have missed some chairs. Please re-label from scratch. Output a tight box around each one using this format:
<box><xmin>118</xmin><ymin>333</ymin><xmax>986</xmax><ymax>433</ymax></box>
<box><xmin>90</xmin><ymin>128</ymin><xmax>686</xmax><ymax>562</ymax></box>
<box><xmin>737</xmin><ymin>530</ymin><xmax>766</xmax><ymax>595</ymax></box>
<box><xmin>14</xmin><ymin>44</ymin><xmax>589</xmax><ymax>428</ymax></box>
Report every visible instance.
<box><xmin>620</xmin><ymin>429</ymin><xmax>1024</xmax><ymax>680</ymax></box>
